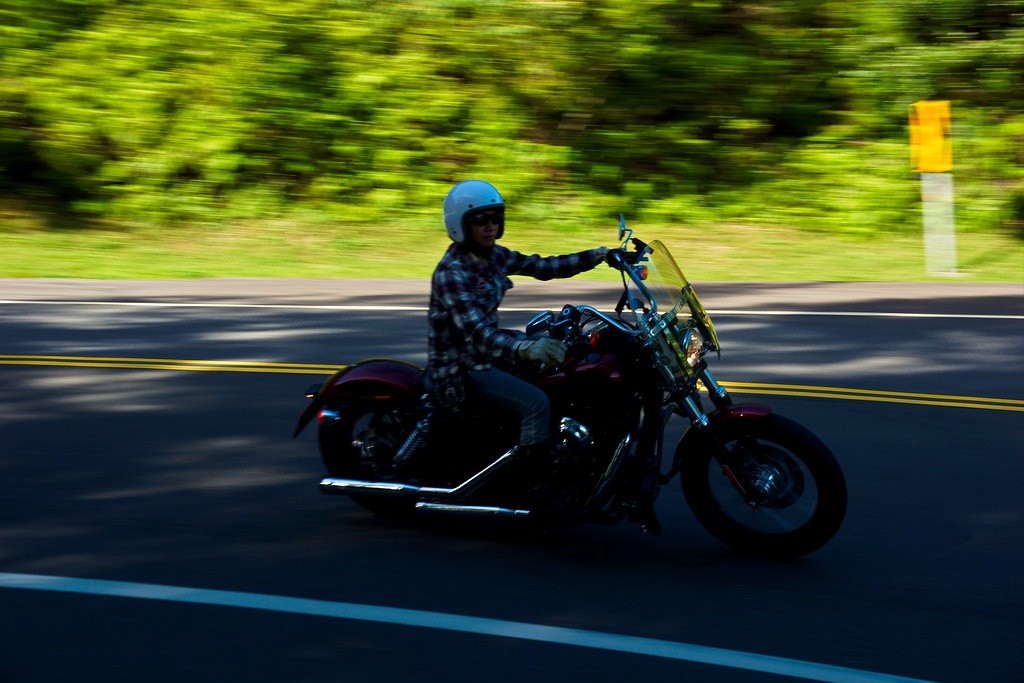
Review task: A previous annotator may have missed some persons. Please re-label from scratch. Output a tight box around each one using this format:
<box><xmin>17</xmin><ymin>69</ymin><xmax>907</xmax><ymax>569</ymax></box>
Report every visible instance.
<box><xmin>428</xmin><ymin>182</ymin><xmax>631</xmax><ymax>521</ymax></box>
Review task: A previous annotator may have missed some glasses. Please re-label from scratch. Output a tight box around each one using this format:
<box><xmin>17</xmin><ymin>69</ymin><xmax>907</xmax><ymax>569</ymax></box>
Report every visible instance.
<box><xmin>467</xmin><ymin>212</ymin><xmax>500</xmax><ymax>227</ymax></box>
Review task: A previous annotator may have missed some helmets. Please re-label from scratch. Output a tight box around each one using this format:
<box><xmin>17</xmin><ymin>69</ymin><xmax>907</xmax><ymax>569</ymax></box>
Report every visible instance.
<box><xmin>444</xmin><ymin>180</ymin><xmax>505</xmax><ymax>242</ymax></box>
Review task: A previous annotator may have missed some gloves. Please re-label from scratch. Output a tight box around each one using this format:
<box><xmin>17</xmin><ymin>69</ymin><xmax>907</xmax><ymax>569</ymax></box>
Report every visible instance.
<box><xmin>519</xmin><ymin>337</ymin><xmax>567</xmax><ymax>366</ymax></box>
<box><xmin>595</xmin><ymin>246</ymin><xmax>622</xmax><ymax>263</ymax></box>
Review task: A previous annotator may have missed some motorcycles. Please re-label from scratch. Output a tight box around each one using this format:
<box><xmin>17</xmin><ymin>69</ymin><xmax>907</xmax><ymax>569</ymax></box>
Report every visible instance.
<box><xmin>292</xmin><ymin>211</ymin><xmax>848</xmax><ymax>563</ymax></box>
